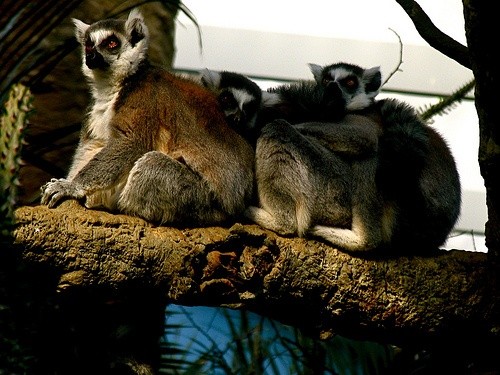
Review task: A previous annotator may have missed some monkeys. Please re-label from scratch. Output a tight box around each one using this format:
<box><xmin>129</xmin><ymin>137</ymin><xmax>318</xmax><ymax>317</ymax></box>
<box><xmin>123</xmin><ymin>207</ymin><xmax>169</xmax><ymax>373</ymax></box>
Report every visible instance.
<box><xmin>39</xmin><ymin>6</ymin><xmax>256</xmax><ymax>228</ymax></box>
<box><xmin>200</xmin><ymin>62</ymin><xmax>463</xmax><ymax>260</ymax></box>
